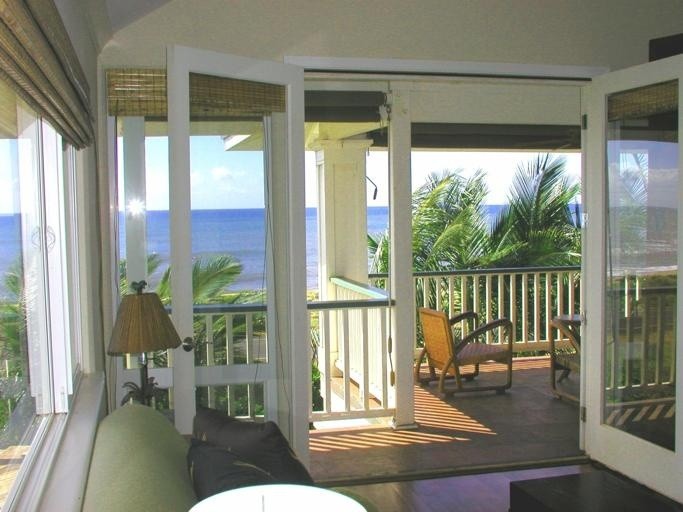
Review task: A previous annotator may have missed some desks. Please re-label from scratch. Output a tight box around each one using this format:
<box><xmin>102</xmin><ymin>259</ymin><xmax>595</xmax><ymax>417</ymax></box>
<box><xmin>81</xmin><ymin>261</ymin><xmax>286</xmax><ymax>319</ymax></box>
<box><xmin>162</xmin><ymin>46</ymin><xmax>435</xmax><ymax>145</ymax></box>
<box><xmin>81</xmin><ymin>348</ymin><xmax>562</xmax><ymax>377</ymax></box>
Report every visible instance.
<box><xmin>556</xmin><ymin>315</ymin><xmax>581</xmax><ymax>383</ymax></box>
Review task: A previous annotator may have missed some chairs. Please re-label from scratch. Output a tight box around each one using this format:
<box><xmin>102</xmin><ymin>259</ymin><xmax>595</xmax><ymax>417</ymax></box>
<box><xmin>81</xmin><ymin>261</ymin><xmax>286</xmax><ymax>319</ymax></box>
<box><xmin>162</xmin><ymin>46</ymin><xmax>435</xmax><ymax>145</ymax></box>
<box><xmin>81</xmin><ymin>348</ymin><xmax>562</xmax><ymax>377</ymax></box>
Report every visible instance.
<box><xmin>416</xmin><ymin>308</ymin><xmax>513</xmax><ymax>397</ymax></box>
<box><xmin>550</xmin><ymin>321</ymin><xmax>580</xmax><ymax>403</ymax></box>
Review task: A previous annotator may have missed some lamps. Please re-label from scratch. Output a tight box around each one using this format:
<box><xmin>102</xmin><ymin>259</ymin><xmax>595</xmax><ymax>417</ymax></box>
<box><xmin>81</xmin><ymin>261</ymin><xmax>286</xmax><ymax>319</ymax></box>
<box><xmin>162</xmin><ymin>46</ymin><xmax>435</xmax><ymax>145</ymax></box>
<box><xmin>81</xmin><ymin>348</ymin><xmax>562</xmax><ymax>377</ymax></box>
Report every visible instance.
<box><xmin>107</xmin><ymin>280</ymin><xmax>180</xmax><ymax>406</ymax></box>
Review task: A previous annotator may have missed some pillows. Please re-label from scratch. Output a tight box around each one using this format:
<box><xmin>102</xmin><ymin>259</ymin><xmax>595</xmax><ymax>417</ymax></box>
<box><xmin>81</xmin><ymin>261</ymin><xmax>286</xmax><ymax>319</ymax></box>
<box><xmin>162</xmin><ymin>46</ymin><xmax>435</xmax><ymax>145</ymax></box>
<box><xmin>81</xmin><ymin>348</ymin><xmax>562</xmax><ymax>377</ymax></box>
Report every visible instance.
<box><xmin>193</xmin><ymin>405</ymin><xmax>313</xmax><ymax>485</ymax></box>
<box><xmin>189</xmin><ymin>439</ymin><xmax>275</xmax><ymax>501</ymax></box>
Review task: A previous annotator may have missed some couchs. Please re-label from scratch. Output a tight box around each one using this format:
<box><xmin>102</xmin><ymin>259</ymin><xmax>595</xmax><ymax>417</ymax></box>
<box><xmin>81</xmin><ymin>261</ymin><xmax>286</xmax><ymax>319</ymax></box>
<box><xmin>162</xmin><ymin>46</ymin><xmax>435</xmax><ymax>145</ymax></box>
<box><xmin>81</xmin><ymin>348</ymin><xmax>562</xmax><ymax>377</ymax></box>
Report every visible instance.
<box><xmin>83</xmin><ymin>405</ymin><xmax>377</xmax><ymax>512</ymax></box>
